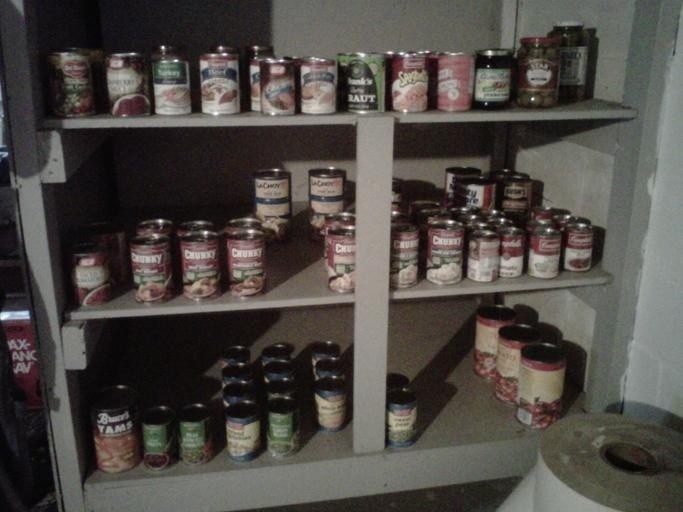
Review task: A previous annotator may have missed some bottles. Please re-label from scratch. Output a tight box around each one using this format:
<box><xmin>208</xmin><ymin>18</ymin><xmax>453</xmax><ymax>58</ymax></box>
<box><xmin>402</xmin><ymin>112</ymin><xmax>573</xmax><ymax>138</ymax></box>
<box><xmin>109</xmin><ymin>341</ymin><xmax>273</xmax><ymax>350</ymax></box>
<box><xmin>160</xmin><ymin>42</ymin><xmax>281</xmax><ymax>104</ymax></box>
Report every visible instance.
<box><xmin>554</xmin><ymin>21</ymin><xmax>592</xmax><ymax>101</ymax></box>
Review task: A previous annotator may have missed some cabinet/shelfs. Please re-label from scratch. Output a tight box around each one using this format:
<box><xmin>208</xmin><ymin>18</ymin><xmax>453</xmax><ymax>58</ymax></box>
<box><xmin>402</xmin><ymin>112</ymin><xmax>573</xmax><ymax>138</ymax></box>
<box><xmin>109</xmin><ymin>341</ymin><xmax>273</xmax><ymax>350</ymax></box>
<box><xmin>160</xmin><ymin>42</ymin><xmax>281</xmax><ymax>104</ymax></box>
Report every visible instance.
<box><xmin>0</xmin><ymin>4</ymin><xmax>659</xmax><ymax>510</ymax></box>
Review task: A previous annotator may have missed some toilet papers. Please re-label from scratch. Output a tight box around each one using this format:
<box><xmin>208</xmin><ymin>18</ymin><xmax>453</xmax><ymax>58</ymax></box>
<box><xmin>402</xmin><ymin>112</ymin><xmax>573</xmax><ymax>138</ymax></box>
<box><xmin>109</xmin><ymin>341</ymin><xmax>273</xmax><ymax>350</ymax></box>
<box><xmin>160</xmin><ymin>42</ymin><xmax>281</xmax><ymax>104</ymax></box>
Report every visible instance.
<box><xmin>491</xmin><ymin>410</ymin><xmax>683</xmax><ymax>512</ymax></box>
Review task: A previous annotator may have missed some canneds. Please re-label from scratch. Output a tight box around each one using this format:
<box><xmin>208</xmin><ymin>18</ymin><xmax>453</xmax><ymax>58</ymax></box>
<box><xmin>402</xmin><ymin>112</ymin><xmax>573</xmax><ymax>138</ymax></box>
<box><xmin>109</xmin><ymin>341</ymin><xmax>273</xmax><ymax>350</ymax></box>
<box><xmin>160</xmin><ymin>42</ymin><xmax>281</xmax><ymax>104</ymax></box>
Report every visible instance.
<box><xmin>307</xmin><ymin>168</ymin><xmax>346</xmax><ymax>230</ymax></box>
<box><xmin>307</xmin><ymin>342</ymin><xmax>338</xmax><ymax>380</ymax></box>
<box><xmin>338</xmin><ymin>51</ymin><xmax>386</xmax><ymax>114</ymax></box>
<box><xmin>263</xmin><ymin>360</ymin><xmax>290</xmax><ymax>384</ymax></box>
<box><xmin>132</xmin><ymin>219</ymin><xmax>171</xmax><ymax>233</ymax></box>
<box><xmin>261</xmin><ymin>344</ymin><xmax>289</xmax><ymax>371</ymax></box>
<box><xmin>266</xmin><ymin>398</ymin><xmax>300</xmax><ymax>458</ymax></box>
<box><xmin>495</xmin><ymin>326</ymin><xmax>538</xmax><ymax>402</ymax></box>
<box><xmin>458</xmin><ymin>214</ymin><xmax>479</xmax><ymax>223</ymax></box>
<box><xmin>251</xmin><ymin>46</ymin><xmax>273</xmax><ymax>110</ymax></box>
<box><xmin>221</xmin><ymin>382</ymin><xmax>251</xmax><ymax>403</ymax></box>
<box><xmin>493</xmin><ymin>169</ymin><xmax>532</xmax><ymax>218</ymax></box>
<box><xmin>420</xmin><ymin>207</ymin><xmax>449</xmax><ymax>227</ymax></box>
<box><xmin>487</xmin><ymin>220</ymin><xmax>514</xmax><ymax>230</ymax></box>
<box><xmin>49</xmin><ymin>50</ymin><xmax>101</xmax><ymax>120</ymax></box>
<box><xmin>553</xmin><ymin>214</ymin><xmax>576</xmax><ymax>226</ymax></box>
<box><xmin>225</xmin><ymin>216</ymin><xmax>258</xmax><ymax>229</ymax></box>
<box><xmin>427</xmin><ymin>51</ymin><xmax>475</xmax><ymax>112</ymax></box>
<box><xmin>391</xmin><ymin>222</ymin><xmax>419</xmax><ymax>287</ymax></box>
<box><xmin>223</xmin><ymin>346</ymin><xmax>249</xmax><ymax>365</ymax></box>
<box><xmin>175</xmin><ymin>402</ymin><xmax>213</xmax><ymax>464</ymax></box>
<box><xmin>498</xmin><ymin>226</ymin><xmax>521</xmax><ymax>279</ymax></box>
<box><xmin>152</xmin><ymin>53</ymin><xmax>189</xmax><ymax>116</ymax></box>
<box><xmin>386</xmin><ymin>373</ymin><xmax>406</xmax><ymax>391</ymax></box>
<box><xmin>529</xmin><ymin>206</ymin><xmax>551</xmax><ymax>221</ymax></box>
<box><xmin>218</xmin><ymin>364</ymin><xmax>256</xmax><ymax>384</ymax></box>
<box><xmin>464</xmin><ymin>219</ymin><xmax>490</xmax><ymax>231</ymax></box>
<box><xmin>562</xmin><ymin>223</ymin><xmax>592</xmax><ymax>273</ymax></box>
<box><xmin>443</xmin><ymin>166</ymin><xmax>480</xmax><ymax>211</ymax></box>
<box><xmin>314</xmin><ymin>357</ymin><xmax>335</xmax><ymax>375</ymax></box>
<box><xmin>409</xmin><ymin>199</ymin><xmax>439</xmax><ymax>224</ymax></box>
<box><xmin>425</xmin><ymin>219</ymin><xmax>467</xmax><ymax>286</ymax></box>
<box><xmin>323</xmin><ymin>229</ymin><xmax>357</xmax><ymax>292</ymax></box>
<box><xmin>468</xmin><ymin>229</ymin><xmax>499</xmax><ymax>283</ymax></box>
<box><xmin>254</xmin><ymin>168</ymin><xmax>289</xmax><ymax>218</ymax></box>
<box><xmin>387</xmin><ymin>389</ymin><xmax>419</xmax><ymax>445</ymax></box>
<box><xmin>551</xmin><ymin>21</ymin><xmax>588</xmax><ymax>103</ymax></box>
<box><xmin>472</xmin><ymin>305</ymin><xmax>516</xmax><ymax>380</ymax></box>
<box><xmin>524</xmin><ymin>220</ymin><xmax>551</xmax><ymax>248</ymax></box>
<box><xmin>260</xmin><ymin>57</ymin><xmax>295</xmax><ymax>117</ymax></box>
<box><xmin>528</xmin><ymin>226</ymin><xmax>561</xmax><ymax>279</ymax></box>
<box><xmin>515</xmin><ymin>344</ymin><xmax>568</xmax><ymax>430</ymax></box>
<box><xmin>224</xmin><ymin>227</ymin><xmax>265</xmax><ymax>299</ymax></box>
<box><xmin>83</xmin><ymin>385</ymin><xmax>144</xmax><ymax>473</ymax></box>
<box><xmin>67</xmin><ymin>244</ymin><xmax>115</xmax><ymax>304</ymax></box>
<box><xmin>223</xmin><ymin>401</ymin><xmax>262</xmax><ymax>461</ymax></box>
<box><xmin>197</xmin><ymin>52</ymin><xmax>239</xmax><ymax>114</ymax></box>
<box><xmin>384</xmin><ymin>51</ymin><xmax>431</xmax><ymax>113</ymax></box>
<box><xmin>142</xmin><ymin>404</ymin><xmax>175</xmax><ymax>471</ymax></box>
<box><xmin>263</xmin><ymin>377</ymin><xmax>294</xmax><ymax>398</ymax></box>
<box><xmin>292</xmin><ymin>57</ymin><xmax>301</xmax><ymax>112</ymax></box>
<box><xmin>391</xmin><ymin>181</ymin><xmax>402</xmax><ymax>222</ymax></box>
<box><xmin>129</xmin><ymin>234</ymin><xmax>173</xmax><ymax>305</ymax></box>
<box><xmin>104</xmin><ymin>52</ymin><xmax>149</xmax><ymax>118</ymax></box>
<box><xmin>312</xmin><ymin>378</ymin><xmax>347</xmax><ymax>432</ymax></box>
<box><xmin>93</xmin><ymin>223</ymin><xmax>131</xmax><ymax>283</ymax></box>
<box><xmin>176</xmin><ymin>220</ymin><xmax>218</xmax><ymax>238</ymax></box>
<box><xmin>322</xmin><ymin>213</ymin><xmax>355</xmax><ymax>257</ymax></box>
<box><xmin>453</xmin><ymin>176</ymin><xmax>497</xmax><ymax>210</ymax></box>
<box><xmin>482</xmin><ymin>216</ymin><xmax>507</xmax><ymax>223</ymax></box>
<box><xmin>179</xmin><ymin>230</ymin><xmax>222</xmax><ymax>303</ymax></box>
<box><xmin>299</xmin><ymin>57</ymin><xmax>337</xmax><ymax>114</ymax></box>
